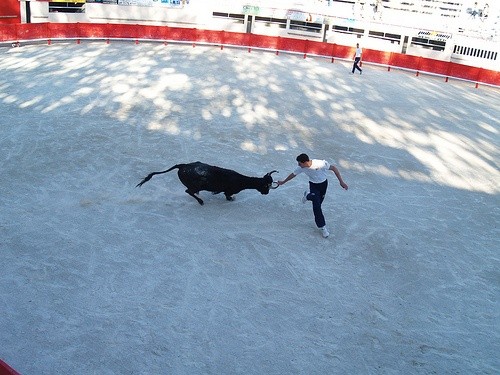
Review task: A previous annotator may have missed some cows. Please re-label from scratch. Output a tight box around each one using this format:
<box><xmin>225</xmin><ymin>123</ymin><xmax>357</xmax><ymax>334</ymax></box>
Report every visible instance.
<box><xmin>136</xmin><ymin>161</ymin><xmax>279</xmax><ymax>206</ymax></box>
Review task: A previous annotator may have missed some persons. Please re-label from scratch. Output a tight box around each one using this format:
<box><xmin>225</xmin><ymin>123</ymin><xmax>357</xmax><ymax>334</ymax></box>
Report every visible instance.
<box><xmin>351</xmin><ymin>42</ymin><xmax>364</xmax><ymax>75</ymax></box>
<box><xmin>278</xmin><ymin>153</ymin><xmax>349</xmax><ymax>238</ymax></box>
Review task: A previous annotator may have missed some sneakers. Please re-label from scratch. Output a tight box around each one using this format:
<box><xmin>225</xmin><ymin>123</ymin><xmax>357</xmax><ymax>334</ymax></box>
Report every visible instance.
<box><xmin>301</xmin><ymin>191</ymin><xmax>310</xmax><ymax>205</ymax></box>
<box><xmin>321</xmin><ymin>226</ymin><xmax>329</xmax><ymax>237</ymax></box>
